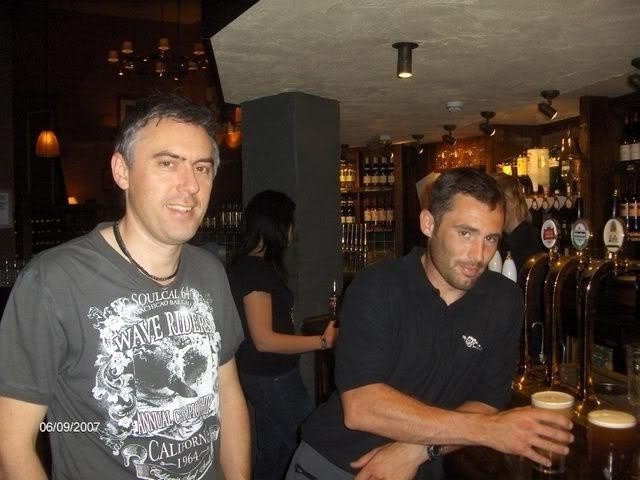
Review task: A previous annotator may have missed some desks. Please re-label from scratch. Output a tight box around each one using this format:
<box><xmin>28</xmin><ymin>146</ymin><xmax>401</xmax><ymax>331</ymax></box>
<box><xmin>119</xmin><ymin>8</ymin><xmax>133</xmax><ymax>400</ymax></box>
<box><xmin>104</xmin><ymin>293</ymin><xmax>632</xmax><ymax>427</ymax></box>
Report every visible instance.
<box><xmin>414</xmin><ymin>412</ymin><xmax>639</xmax><ymax>480</ymax></box>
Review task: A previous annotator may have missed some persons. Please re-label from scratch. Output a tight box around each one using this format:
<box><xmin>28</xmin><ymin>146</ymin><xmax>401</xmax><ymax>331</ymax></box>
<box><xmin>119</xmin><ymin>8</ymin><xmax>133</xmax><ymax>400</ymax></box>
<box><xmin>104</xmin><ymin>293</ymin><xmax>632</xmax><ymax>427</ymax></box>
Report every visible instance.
<box><xmin>284</xmin><ymin>166</ymin><xmax>574</xmax><ymax>480</ymax></box>
<box><xmin>493</xmin><ymin>173</ymin><xmax>548</xmax><ymax>273</ymax></box>
<box><xmin>225</xmin><ymin>190</ymin><xmax>342</xmax><ymax>479</ymax></box>
<box><xmin>0</xmin><ymin>92</ymin><xmax>254</xmax><ymax>479</ymax></box>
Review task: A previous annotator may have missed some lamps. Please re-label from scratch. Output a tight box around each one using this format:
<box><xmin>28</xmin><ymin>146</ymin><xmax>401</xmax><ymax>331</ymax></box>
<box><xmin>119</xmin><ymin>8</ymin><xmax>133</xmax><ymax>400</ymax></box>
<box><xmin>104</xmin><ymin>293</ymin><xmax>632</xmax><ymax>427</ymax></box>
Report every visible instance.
<box><xmin>442</xmin><ymin>124</ymin><xmax>457</xmax><ymax>145</ymax></box>
<box><xmin>480</xmin><ymin>111</ymin><xmax>496</xmax><ymax>136</ymax></box>
<box><xmin>627</xmin><ymin>57</ymin><xmax>640</xmax><ymax>92</ymax></box>
<box><xmin>34</xmin><ymin>129</ymin><xmax>59</xmax><ymax>158</ymax></box>
<box><xmin>539</xmin><ymin>89</ymin><xmax>560</xmax><ymax>121</ymax></box>
<box><xmin>105</xmin><ymin>1</ymin><xmax>210</xmax><ymax>80</ymax></box>
<box><xmin>392</xmin><ymin>41</ymin><xmax>420</xmax><ymax>80</ymax></box>
<box><xmin>413</xmin><ymin>134</ymin><xmax>426</xmax><ymax>155</ymax></box>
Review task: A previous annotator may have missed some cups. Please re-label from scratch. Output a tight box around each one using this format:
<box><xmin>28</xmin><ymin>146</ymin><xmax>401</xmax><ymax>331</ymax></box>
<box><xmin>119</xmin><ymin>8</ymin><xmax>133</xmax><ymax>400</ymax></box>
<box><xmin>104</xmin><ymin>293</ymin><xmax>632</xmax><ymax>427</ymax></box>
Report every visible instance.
<box><xmin>585</xmin><ymin>410</ymin><xmax>638</xmax><ymax>479</ymax></box>
<box><xmin>528</xmin><ymin>390</ymin><xmax>576</xmax><ymax>472</ymax></box>
<box><xmin>435</xmin><ymin>147</ymin><xmax>487</xmax><ymax>170</ymax></box>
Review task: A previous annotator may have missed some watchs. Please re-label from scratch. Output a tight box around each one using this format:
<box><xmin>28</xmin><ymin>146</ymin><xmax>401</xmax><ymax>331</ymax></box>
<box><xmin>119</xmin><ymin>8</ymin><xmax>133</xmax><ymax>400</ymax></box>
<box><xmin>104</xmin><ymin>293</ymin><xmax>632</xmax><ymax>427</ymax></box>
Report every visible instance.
<box><xmin>425</xmin><ymin>445</ymin><xmax>445</xmax><ymax>464</ymax></box>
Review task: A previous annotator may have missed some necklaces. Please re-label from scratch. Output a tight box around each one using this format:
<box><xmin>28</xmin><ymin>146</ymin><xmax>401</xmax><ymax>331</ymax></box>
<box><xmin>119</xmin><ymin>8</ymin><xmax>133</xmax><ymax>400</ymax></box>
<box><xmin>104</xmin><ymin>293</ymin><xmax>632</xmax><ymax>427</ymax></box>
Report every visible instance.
<box><xmin>112</xmin><ymin>218</ymin><xmax>180</xmax><ymax>281</ymax></box>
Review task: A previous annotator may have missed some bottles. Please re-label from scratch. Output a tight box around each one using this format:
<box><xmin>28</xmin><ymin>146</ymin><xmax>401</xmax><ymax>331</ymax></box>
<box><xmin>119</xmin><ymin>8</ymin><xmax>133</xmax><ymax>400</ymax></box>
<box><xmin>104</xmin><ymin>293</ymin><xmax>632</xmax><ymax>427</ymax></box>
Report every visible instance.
<box><xmin>188</xmin><ymin>203</ymin><xmax>244</xmax><ymax>254</ymax></box>
<box><xmin>363</xmin><ymin>152</ymin><xmax>396</xmax><ymax>186</ymax></box>
<box><xmin>362</xmin><ymin>190</ymin><xmax>392</xmax><ymax>233</ymax></box>
<box><xmin>488</xmin><ymin>249</ymin><xmax>503</xmax><ymax>273</ymax></box>
<box><xmin>338</xmin><ymin>161</ymin><xmax>356</xmax><ymax>223</ymax></box>
<box><xmin>503</xmin><ymin>250</ymin><xmax>518</xmax><ymax>284</ymax></box>
<box><xmin>608</xmin><ymin>188</ymin><xmax>618</xmax><ymax>221</ymax></box>
<box><xmin>339</xmin><ymin>222</ymin><xmax>369</xmax><ymax>272</ymax></box>
<box><xmin>557</xmin><ymin>132</ymin><xmax>581</xmax><ymax>196</ymax></box>
<box><xmin>517</xmin><ymin>154</ymin><xmax>529</xmax><ymax>195</ymax></box>
<box><xmin>327</xmin><ymin>279</ymin><xmax>341</xmax><ymax>323</ymax></box>
<box><xmin>618</xmin><ymin>111</ymin><xmax>640</xmax><ymax>235</ymax></box>
<box><xmin>503</xmin><ymin>163</ymin><xmax>513</xmax><ymax>197</ymax></box>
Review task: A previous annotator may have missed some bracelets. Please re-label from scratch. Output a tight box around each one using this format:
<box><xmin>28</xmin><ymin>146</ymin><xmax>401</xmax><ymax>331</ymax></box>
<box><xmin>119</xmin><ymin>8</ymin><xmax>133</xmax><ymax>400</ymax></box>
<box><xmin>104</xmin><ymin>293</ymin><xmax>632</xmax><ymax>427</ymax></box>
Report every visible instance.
<box><xmin>319</xmin><ymin>335</ymin><xmax>326</xmax><ymax>349</ymax></box>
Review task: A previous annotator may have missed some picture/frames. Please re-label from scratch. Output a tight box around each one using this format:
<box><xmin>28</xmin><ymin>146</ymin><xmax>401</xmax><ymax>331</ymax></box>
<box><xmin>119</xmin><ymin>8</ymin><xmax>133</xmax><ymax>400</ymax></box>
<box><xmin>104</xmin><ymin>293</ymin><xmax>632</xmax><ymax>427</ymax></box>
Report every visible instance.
<box><xmin>0</xmin><ymin>186</ymin><xmax>15</xmax><ymax>230</ymax></box>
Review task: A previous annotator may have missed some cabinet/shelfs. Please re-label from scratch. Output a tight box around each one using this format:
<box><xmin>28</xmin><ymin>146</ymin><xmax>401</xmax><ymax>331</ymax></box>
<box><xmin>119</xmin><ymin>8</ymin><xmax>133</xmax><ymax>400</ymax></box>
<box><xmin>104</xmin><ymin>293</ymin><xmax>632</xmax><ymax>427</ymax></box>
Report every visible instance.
<box><xmin>341</xmin><ymin>144</ymin><xmax>415</xmax><ymax>259</ymax></box>
